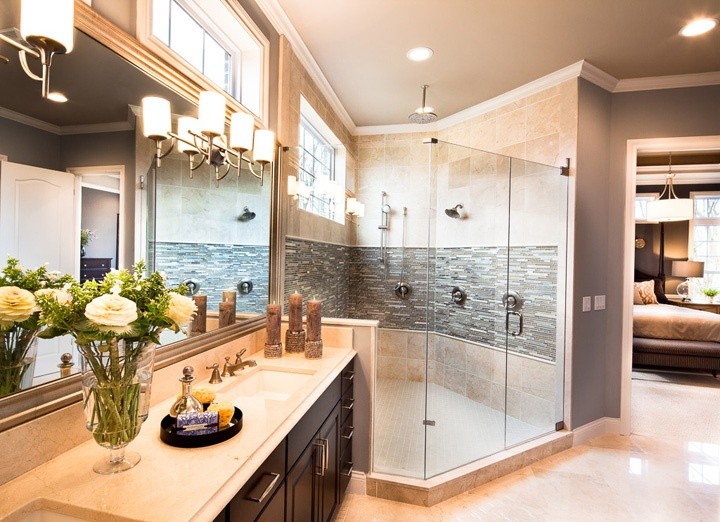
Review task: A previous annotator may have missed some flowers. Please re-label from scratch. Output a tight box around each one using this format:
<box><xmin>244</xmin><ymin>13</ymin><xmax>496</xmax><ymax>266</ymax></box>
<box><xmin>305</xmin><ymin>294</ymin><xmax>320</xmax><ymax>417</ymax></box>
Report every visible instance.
<box><xmin>33</xmin><ymin>260</ymin><xmax>200</xmax><ymax>447</ymax></box>
<box><xmin>81</xmin><ymin>228</ymin><xmax>95</xmax><ymax>246</ymax></box>
<box><xmin>0</xmin><ymin>256</ymin><xmax>81</xmax><ymax>399</ymax></box>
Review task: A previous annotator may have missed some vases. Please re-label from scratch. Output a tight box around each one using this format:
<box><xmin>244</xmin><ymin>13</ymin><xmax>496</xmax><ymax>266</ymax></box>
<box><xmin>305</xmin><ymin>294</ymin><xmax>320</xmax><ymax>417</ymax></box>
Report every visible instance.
<box><xmin>81</xmin><ymin>244</ymin><xmax>85</xmax><ymax>257</ymax></box>
<box><xmin>76</xmin><ymin>341</ymin><xmax>155</xmax><ymax>475</ymax></box>
<box><xmin>0</xmin><ymin>333</ymin><xmax>38</xmax><ymax>398</ymax></box>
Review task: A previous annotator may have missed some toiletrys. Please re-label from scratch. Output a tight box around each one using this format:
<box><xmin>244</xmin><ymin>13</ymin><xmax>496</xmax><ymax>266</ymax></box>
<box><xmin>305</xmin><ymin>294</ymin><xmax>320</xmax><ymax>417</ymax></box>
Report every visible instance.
<box><xmin>169</xmin><ymin>365</ymin><xmax>204</xmax><ymax>418</ymax></box>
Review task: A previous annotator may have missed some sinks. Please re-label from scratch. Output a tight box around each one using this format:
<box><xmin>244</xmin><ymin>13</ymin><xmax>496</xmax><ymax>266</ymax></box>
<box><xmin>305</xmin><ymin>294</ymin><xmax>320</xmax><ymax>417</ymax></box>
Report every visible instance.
<box><xmin>217</xmin><ymin>365</ymin><xmax>316</xmax><ymax>402</ymax></box>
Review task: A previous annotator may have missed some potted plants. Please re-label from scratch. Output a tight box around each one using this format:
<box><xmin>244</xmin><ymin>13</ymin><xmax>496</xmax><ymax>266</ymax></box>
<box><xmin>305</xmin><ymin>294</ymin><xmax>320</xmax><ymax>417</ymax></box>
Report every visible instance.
<box><xmin>703</xmin><ymin>289</ymin><xmax>718</xmax><ymax>303</ymax></box>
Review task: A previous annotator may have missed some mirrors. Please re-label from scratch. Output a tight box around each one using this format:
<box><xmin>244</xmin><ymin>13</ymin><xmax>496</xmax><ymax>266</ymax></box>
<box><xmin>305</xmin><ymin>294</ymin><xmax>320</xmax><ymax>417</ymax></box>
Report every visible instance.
<box><xmin>0</xmin><ymin>0</ymin><xmax>280</xmax><ymax>430</ymax></box>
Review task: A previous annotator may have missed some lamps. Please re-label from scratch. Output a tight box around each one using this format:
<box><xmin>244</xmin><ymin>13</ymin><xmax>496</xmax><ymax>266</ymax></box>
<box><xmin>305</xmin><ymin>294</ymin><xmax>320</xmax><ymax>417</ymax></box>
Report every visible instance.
<box><xmin>0</xmin><ymin>0</ymin><xmax>75</xmax><ymax>97</ymax></box>
<box><xmin>188</xmin><ymin>89</ymin><xmax>274</xmax><ymax>185</ymax></box>
<box><xmin>141</xmin><ymin>96</ymin><xmax>227</xmax><ymax>188</ymax></box>
<box><xmin>645</xmin><ymin>151</ymin><xmax>693</xmax><ymax>223</ymax></box>
<box><xmin>672</xmin><ymin>260</ymin><xmax>704</xmax><ymax>301</ymax></box>
<box><xmin>288</xmin><ymin>174</ymin><xmax>365</xmax><ymax>223</ymax></box>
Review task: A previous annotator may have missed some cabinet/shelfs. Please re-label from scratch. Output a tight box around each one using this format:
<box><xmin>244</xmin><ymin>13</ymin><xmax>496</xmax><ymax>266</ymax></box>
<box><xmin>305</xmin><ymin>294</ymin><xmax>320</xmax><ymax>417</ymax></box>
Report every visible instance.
<box><xmin>80</xmin><ymin>258</ymin><xmax>112</xmax><ymax>287</ymax></box>
<box><xmin>214</xmin><ymin>361</ymin><xmax>356</xmax><ymax>522</ymax></box>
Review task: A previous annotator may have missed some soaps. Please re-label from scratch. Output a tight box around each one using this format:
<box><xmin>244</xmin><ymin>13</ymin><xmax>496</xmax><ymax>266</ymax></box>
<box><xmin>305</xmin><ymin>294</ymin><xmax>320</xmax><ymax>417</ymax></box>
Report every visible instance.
<box><xmin>177</xmin><ymin>411</ymin><xmax>219</xmax><ymax>436</ymax></box>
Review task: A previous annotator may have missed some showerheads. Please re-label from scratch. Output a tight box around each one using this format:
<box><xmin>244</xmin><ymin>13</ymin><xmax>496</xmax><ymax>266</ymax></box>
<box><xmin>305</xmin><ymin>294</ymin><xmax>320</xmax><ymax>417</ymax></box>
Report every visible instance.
<box><xmin>381</xmin><ymin>204</ymin><xmax>391</xmax><ymax>226</ymax></box>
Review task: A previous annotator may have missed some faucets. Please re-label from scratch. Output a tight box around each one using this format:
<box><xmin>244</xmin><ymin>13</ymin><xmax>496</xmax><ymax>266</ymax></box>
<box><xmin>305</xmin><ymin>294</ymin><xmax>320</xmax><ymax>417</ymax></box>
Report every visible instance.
<box><xmin>221</xmin><ymin>356</ymin><xmax>257</xmax><ymax>377</ymax></box>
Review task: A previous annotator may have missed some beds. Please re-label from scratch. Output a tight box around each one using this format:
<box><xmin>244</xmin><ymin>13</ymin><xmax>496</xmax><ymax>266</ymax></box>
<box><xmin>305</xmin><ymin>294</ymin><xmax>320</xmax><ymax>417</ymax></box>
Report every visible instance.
<box><xmin>630</xmin><ymin>267</ymin><xmax>720</xmax><ymax>377</ymax></box>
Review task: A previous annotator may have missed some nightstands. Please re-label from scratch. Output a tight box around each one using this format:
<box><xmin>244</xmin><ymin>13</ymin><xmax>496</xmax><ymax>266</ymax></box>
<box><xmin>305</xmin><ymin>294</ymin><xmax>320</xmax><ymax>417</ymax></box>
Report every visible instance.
<box><xmin>668</xmin><ymin>298</ymin><xmax>720</xmax><ymax>314</ymax></box>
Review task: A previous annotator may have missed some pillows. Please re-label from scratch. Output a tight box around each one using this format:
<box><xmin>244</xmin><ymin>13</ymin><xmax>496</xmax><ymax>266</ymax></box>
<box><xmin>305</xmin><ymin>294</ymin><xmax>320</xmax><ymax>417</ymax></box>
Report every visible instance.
<box><xmin>634</xmin><ymin>278</ymin><xmax>670</xmax><ymax>305</ymax></box>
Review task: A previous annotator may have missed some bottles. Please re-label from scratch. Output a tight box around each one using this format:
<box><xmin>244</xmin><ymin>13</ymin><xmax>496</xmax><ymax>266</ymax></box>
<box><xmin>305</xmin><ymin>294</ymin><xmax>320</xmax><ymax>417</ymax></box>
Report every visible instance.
<box><xmin>169</xmin><ymin>366</ymin><xmax>204</xmax><ymax>419</ymax></box>
<box><xmin>56</xmin><ymin>352</ymin><xmax>75</xmax><ymax>378</ymax></box>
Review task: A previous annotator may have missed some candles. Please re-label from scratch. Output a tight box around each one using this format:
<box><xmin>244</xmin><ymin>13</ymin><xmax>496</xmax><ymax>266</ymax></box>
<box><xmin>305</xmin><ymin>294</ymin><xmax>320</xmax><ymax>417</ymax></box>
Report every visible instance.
<box><xmin>267</xmin><ymin>290</ymin><xmax>323</xmax><ymax>346</ymax></box>
<box><xmin>191</xmin><ymin>290</ymin><xmax>235</xmax><ymax>335</ymax></box>
